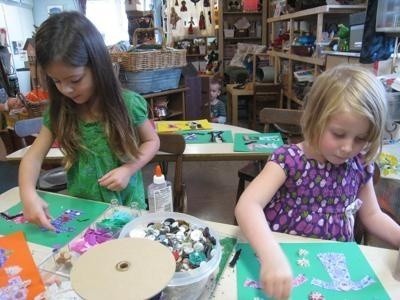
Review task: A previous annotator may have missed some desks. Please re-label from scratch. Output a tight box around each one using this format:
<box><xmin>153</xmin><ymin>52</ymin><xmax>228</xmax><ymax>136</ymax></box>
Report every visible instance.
<box><xmin>227</xmin><ymin>82</ymin><xmax>282</xmax><ymax>126</ymax></box>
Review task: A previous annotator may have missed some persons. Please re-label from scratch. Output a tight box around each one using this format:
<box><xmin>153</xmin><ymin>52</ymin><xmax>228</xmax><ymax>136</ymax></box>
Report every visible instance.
<box><xmin>17</xmin><ymin>12</ymin><xmax>162</xmax><ymax>233</ymax></box>
<box><xmin>1</xmin><ymin>98</ymin><xmax>20</xmax><ymax>162</ymax></box>
<box><xmin>234</xmin><ymin>64</ymin><xmax>400</xmax><ymax>300</ymax></box>
<box><xmin>209</xmin><ymin>78</ymin><xmax>228</xmax><ymax>124</ymax></box>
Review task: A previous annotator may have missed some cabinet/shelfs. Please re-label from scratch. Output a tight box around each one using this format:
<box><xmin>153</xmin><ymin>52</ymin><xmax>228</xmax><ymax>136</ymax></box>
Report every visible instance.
<box><xmin>136</xmin><ymin>87</ymin><xmax>191</xmax><ymax>121</ymax></box>
<box><xmin>218</xmin><ymin>0</ymin><xmax>367</xmax><ymax>109</ymax></box>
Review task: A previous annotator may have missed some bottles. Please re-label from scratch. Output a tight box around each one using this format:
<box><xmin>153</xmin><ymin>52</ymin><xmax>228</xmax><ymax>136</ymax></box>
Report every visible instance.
<box><xmin>146</xmin><ymin>165</ymin><xmax>174</xmax><ymax>213</ymax></box>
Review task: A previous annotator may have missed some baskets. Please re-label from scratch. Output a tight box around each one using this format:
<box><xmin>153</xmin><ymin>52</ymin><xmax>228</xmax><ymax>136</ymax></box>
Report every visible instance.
<box><xmin>110</xmin><ymin>27</ymin><xmax>187</xmax><ymax>71</ymax></box>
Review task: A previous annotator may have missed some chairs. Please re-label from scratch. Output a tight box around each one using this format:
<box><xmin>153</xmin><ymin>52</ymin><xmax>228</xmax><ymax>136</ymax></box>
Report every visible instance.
<box><xmin>235</xmin><ymin>108</ymin><xmax>305</xmax><ymax>225</ymax></box>
<box><xmin>252</xmin><ymin>84</ymin><xmax>282</xmax><ymax>127</ymax></box>
